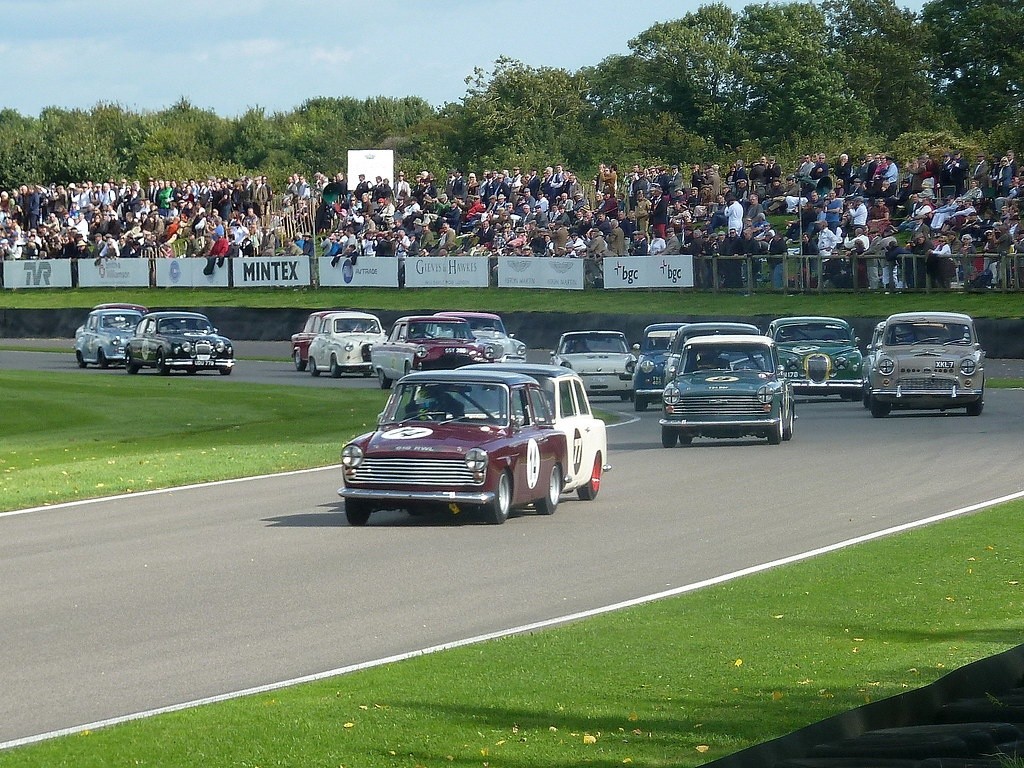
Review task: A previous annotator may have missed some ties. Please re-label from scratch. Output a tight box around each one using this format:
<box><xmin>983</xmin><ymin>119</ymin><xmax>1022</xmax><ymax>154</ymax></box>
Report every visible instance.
<box><xmin>489</xmin><ymin>204</ymin><xmax>494</xmax><ymax>210</ymax></box>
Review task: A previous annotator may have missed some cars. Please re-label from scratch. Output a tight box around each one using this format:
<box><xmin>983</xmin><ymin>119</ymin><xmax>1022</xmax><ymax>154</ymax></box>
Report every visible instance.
<box><xmin>765</xmin><ymin>316</ymin><xmax>864</xmax><ymax>402</ymax></box>
<box><xmin>73</xmin><ymin>309</ymin><xmax>143</xmax><ymax>369</ymax></box>
<box><xmin>662</xmin><ymin>323</ymin><xmax>765</xmax><ymax>416</ymax></box>
<box><xmin>93</xmin><ymin>304</ymin><xmax>150</xmax><ymax>316</ymax></box>
<box><xmin>551</xmin><ymin>331</ymin><xmax>638</xmax><ymax>403</ymax></box>
<box><xmin>861</xmin><ymin>312</ymin><xmax>988</xmax><ymax>417</ymax></box>
<box><xmin>339</xmin><ymin>363</ymin><xmax>614</xmax><ymax>525</ymax></box>
<box><xmin>308</xmin><ymin>312</ymin><xmax>389</xmax><ymax>378</ymax></box>
<box><xmin>371</xmin><ymin>315</ymin><xmax>494</xmax><ymax>389</ymax></box>
<box><xmin>290</xmin><ymin>311</ymin><xmax>348</xmax><ymax>371</ymax></box>
<box><xmin>626</xmin><ymin>323</ymin><xmax>686</xmax><ymax>410</ymax></box>
<box><xmin>125</xmin><ymin>311</ymin><xmax>236</xmax><ymax>375</ymax></box>
<box><xmin>660</xmin><ymin>335</ymin><xmax>794</xmax><ymax>447</ymax></box>
<box><xmin>425</xmin><ymin>311</ymin><xmax>527</xmax><ymax>364</ymax></box>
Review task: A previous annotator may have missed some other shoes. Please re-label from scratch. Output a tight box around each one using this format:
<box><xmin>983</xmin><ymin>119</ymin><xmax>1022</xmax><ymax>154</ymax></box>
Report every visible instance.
<box><xmin>885</xmin><ymin>291</ymin><xmax>890</xmax><ymax>295</ymax></box>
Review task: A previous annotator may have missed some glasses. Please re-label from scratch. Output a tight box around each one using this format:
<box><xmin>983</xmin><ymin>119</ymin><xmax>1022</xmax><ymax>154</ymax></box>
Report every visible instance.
<box><xmin>633</xmin><ymin>234</ymin><xmax>637</xmax><ymax>236</ymax></box>
<box><xmin>987</xmin><ymin>234</ymin><xmax>992</xmax><ymax>236</ymax></box>
<box><xmin>730</xmin><ymin>232</ymin><xmax>735</xmax><ymax>234</ymax></box>
<box><xmin>964</xmin><ymin>238</ymin><xmax>970</xmax><ymax>240</ymax></box>
<box><xmin>666</xmin><ymin>231</ymin><xmax>673</xmax><ymax>233</ymax></box>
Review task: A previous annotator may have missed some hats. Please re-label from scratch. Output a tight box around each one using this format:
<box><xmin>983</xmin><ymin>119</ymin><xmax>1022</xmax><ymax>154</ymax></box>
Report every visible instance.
<box><xmin>914</xmin><ymin>232</ymin><xmax>924</xmax><ymax>240</ymax></box>
<box><xmin>498</xmin><ymin>194</ymin><xmax>506</xmax><ymax>199</ymax></box>
<box><xmin>189</xmin><ymin>232</ymin><xmax>195</xmax><ymax>236</ymax></box>
<box><xmin>815</xmin><ymin>220</ymin><xmax>828</xmax><ymax>226</ymax></box>
<box><xmin>571</xmin><ymin>233</ymin><xmax>578</xmax><ymax>236</ymax></box>
<box><xmin>758</xmin><ymin>213</ymin><xmax>766</xmax><ymax>221</ymax></box>
<box><xmin>94</xmin><ymin>233</ymin><xmax>102</xmax><ymax>236</ymax></box>
<box><xmin>204</xmin><ymin>233</ymin><xmax>212</xmax><ymax>236</ymax></box>
<box><xmin>947</xmin><ymin>231</ymin><xmax>956</xmax><ymax>235</ymax></box>
<box><xmin>522</xmin><ymin>245</ymin><xmax>532</xmax><ymax>250</ymax></box>
<box><xmin>976</xmin><ymin>153</ymin><xmax>985</xmax><ymax>156</ymax></box>
<box><xmin>666</xmin><ymin>228</ymin><xmax>674</xmax><ymax>231</ymax></box>
<box><xmin>302</xmin><ymin>232</ymin><xmax>312</xmax><ymax>238</ymax></box>
<box><xmin>597</xmin><ymin>231</ymin><xmax>605</xmax><ymax>237</ymax></box>
<box><xmin>489</xmin><ymin>195</ymin><xmax>496</xmax><ymax>199</ymax></box>
<box><xmin>412</xmin><ymin>218</ymin><xmax>422</xmax><ymax>226</ymax></box>
<box><xmin>1</xmin><ymin>239</ymin><xmax>8</xmax><ymax>243</ymax></box>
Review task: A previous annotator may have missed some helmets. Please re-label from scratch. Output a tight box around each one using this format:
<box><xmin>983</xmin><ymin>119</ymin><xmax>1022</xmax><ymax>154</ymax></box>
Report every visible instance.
<box><xmin>895</xmin><ymin>325</ymin><xmax>916</xmax><ymax>344</ymax></box>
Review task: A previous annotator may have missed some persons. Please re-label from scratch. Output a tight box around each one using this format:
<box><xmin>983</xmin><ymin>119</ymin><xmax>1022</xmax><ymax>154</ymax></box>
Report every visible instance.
<box><xmin>0</xmin><ymin>150</ymin><xmax>1024</xmax><ymax>292</ymax></box>
<box><xmin>893</xmin><ymin>324</ymin><xmax>916</xmax><ymax>343</ymax></box>
<box><xmin>409</xmin><ymin>389</ymin><xmax>438</xmax><ymax>420</ymax></box>
<box><xmin>693</xmin><ymin>350</ymin><xmax>720</xmax><ymax>370</ymax></box>
<box><xmin>413</xmin><ymin>325</ymin><xmax>427</xmax><ymax>338</ymax></box>
<box><xmin>570</xmin><ymin>339</ymin><xmax>590</xmax><ymax>352</ymax></box>
<box><xmin>780</xmin><ymin>329</ymin><xmax>794</xmax><ymax>341</ymax></box>
